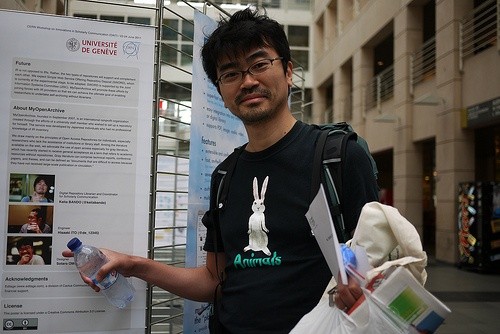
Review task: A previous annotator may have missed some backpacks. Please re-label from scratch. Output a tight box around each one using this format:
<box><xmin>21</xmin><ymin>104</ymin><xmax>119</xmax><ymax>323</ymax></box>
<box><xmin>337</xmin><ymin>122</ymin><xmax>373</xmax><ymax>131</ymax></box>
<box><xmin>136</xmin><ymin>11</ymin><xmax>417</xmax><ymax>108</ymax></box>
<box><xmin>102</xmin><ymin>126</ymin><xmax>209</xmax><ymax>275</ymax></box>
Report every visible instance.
<box><xmin>216</xmin><ymin>122</ymin><xmax>379</xmax><ymax>244</ymax></box>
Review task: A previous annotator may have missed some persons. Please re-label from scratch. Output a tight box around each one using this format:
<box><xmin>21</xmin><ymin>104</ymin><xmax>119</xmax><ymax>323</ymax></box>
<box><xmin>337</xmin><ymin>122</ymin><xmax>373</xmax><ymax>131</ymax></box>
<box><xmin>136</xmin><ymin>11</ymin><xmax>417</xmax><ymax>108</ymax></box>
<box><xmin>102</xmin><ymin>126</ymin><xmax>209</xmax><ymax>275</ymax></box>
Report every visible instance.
<box><xmin>20</xmin><ymin>176</ymin><xmax>54</xmax><ymax>203</ymax></box>
<box><xmin>15</xmin><ymin>238</ymin><xmax>46</xmax><ymax>265</ymax></box>
<box><xmin>63</xmin><ymin>9</ymin><xmax>379</xmax><ymax>334</ymax></box>
<box><xmin>19</xmin><ymin>208</ymin><xmax>52</xmax><ymax>234</ymax></box>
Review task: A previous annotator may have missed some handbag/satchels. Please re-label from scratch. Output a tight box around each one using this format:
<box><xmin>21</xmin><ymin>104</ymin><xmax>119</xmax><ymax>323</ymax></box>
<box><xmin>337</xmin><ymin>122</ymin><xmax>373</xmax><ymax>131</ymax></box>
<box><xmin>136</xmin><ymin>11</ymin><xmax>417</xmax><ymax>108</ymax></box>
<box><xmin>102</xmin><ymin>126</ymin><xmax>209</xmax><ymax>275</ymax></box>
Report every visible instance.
<box><xmin>290</xmin><ymin>287</ymin><xmax>424</xmax><ymax>334</ymax></box>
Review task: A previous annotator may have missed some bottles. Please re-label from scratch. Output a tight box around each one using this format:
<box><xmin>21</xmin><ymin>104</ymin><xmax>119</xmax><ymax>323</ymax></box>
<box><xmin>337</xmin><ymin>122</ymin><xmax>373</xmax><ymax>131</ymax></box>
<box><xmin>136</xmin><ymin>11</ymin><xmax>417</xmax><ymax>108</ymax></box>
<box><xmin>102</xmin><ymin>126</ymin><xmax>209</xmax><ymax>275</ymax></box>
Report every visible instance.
<box><xmin>67</xmin><ymin>237</ymin><xmax>136</xmax><ymax>308</ymax></box>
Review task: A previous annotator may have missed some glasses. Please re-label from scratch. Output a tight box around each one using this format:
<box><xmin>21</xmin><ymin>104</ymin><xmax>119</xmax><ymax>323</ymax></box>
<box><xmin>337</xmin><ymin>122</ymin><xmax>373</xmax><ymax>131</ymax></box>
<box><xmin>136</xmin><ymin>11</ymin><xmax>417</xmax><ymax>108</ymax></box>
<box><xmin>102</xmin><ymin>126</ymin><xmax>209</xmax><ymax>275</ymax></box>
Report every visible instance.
<box><xmin>216</xmin><ymin>57</ymin><xmax>283</xmax><ymax>85</ymax></box>
